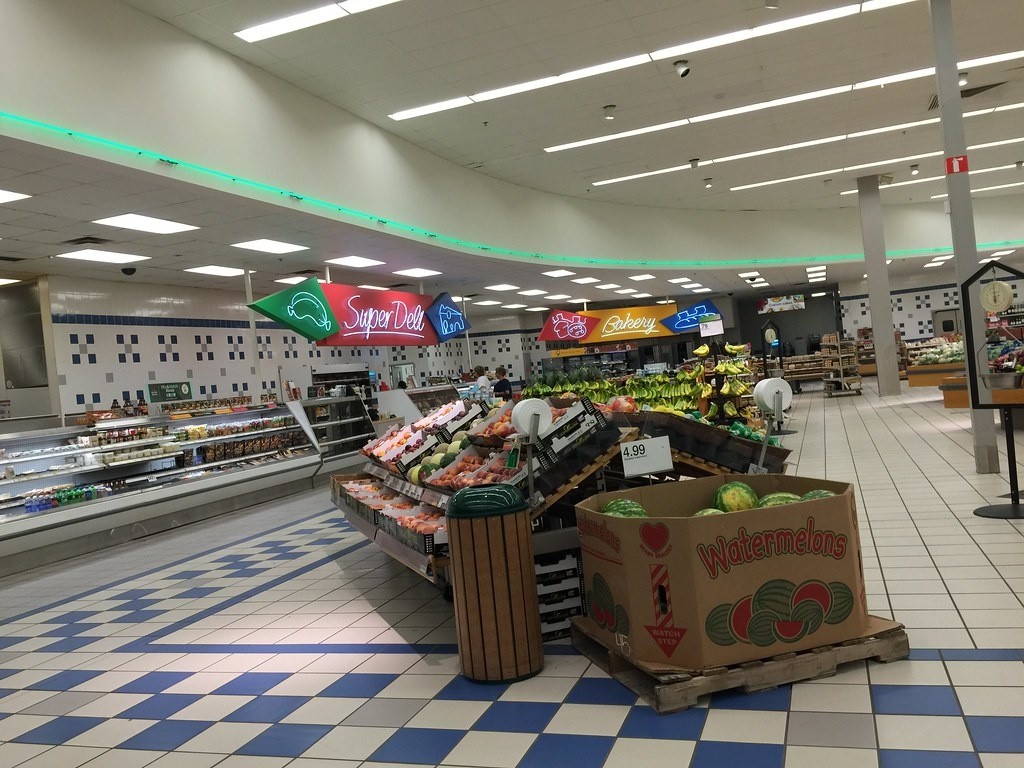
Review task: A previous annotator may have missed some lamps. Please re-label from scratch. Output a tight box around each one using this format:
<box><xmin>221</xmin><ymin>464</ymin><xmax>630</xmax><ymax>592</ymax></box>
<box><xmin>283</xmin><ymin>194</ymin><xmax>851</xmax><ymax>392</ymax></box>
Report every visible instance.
<box><xmin>690</xmin><ymin>159</ymin><xmax>699</xmax><ymax>170</ymax></box>
<box><xmin>604</xmin><ymin>106</ymin><xmax>616</xmax><ymax>120</ymax></box>
<box><xmin>880</xmin><ymin>176</ymin><xmax>893</xmax><ymax>185</ymax></box>
<box><xmin>911</xmin><ymin>165</ymin><xmax>919</xmax><ymax>175</ymax></box>
<box><xmin>705</xmin><ymin>178</ymin><xmax>712</xmax><ymax>188</ymax></box>
<box><xmin>675</xmin><ymin>61</ymin><xmax>690</xmax><ymax>78</ymax></box>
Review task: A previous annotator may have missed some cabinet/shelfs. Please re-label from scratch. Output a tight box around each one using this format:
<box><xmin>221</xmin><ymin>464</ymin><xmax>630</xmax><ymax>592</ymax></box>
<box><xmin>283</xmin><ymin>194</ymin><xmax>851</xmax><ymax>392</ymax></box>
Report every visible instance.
<box><xmin>858</xmin><ymin>337</ymin><xmax>1024</xmax><ymax>408</ymax></box>
<box><xmin>820</xmin><ymin>331</ymin><xmax>863</xmax><ymax>398</ymax></box>
<box><xmin>0</xmin><ymin>385</ymin><xmax>459</xmax><ymax>525</ymax></box>
<box><xmin>691</xmin><ymin>355</ymin><xmax>820</xmax><ymax>431</ymax></box>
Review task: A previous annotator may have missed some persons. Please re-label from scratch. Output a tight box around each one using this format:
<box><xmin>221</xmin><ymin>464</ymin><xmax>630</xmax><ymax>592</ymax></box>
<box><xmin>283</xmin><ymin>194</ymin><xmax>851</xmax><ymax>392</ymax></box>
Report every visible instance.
<box><xmin>474</xmin><ymin>366</ymin><xmax>491</xmax><ymax>394</ymax></box>
<box><xmin>493</xmin><ymin>367</ymin><xmax>512</xmax><ymax>401</ymax></box>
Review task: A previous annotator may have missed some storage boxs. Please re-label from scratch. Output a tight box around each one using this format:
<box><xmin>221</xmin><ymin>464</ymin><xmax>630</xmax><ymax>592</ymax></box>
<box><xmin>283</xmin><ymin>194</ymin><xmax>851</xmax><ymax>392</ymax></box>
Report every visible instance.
<box><xmin>330</xmin><ymin>400</ymin><xmax>621</xmax><ymax>558</ymax></box>
<box><xmin>574</xmin><ymin>476</ymin><xmax>867</xmax><ymax>670</ymax></box>
<box><xmin>532</xmin><ymin>552</ymin><xmax>585</xmax><ymax>642</ymax></box>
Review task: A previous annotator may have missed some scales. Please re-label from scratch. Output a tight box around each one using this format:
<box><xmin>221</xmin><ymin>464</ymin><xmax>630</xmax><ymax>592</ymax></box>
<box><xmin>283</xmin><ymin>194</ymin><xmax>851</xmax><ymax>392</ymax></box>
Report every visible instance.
<box><xmin>764</xmin><ymin>328</ymin><xmax>785</xmax><ymax>377</ymax></box>
<box><xmin>976</xmin><ymin>276</ymin><xmax>1024</xmax><ymax>390</ymax></box>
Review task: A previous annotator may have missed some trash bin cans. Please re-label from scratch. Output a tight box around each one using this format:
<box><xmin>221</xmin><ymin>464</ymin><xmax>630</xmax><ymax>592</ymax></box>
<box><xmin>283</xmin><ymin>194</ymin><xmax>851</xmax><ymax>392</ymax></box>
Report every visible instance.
<box><xmin>444</xmin><ymin>483</ymin><xmax>546</xmax><ymax>684</ymax></box>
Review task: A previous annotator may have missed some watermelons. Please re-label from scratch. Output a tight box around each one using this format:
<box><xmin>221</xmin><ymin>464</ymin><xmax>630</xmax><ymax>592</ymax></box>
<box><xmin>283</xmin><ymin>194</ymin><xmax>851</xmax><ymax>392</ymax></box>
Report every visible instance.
<box><xmin>754</xmin><ymin>492</ymin><xmax>801</xmax><ymax>508</ymax></box>
<box><xmin>693</xmin><ymin>508</ymin><xmax>725</xmax><ymax>516</ymax></box>
<box><xmin>713</xmin><ymin>480</ymin><xmax>758</xmax><ymax>512</ymax></box>
<box><xmin>799</xmin><ymin>490</ymin><xmax>836</xmax><ymax>502</ymax></box>
<box><xmin>600</xmin><ymin>500</ymin><xmax>649</xmax><ymax>518</ymax></box>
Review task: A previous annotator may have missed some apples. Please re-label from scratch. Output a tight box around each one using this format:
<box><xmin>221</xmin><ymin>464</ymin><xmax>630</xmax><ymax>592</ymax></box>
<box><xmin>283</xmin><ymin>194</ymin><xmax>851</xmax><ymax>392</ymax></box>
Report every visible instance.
<box><xmin>344</xmin><ymin>430</ymin><xmax>447</xmax><ymax>533</ymax></box>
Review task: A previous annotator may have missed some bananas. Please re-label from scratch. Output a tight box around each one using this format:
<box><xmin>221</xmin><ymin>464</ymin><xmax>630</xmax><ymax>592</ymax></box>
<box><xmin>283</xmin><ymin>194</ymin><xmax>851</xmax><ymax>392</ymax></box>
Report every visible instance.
<box><xmin>521</xmin><ymin>342</ymin><xmax>754</xmax><ymax>418</ymax></box>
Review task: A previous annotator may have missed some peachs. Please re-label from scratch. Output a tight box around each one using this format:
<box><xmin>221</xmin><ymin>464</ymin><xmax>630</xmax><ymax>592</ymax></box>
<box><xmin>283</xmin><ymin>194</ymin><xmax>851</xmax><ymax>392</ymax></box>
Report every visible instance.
<box><xmin>430</xmin><ymin>407</ymin><xmax>572</xmax><ymax>490</ymax></box>
